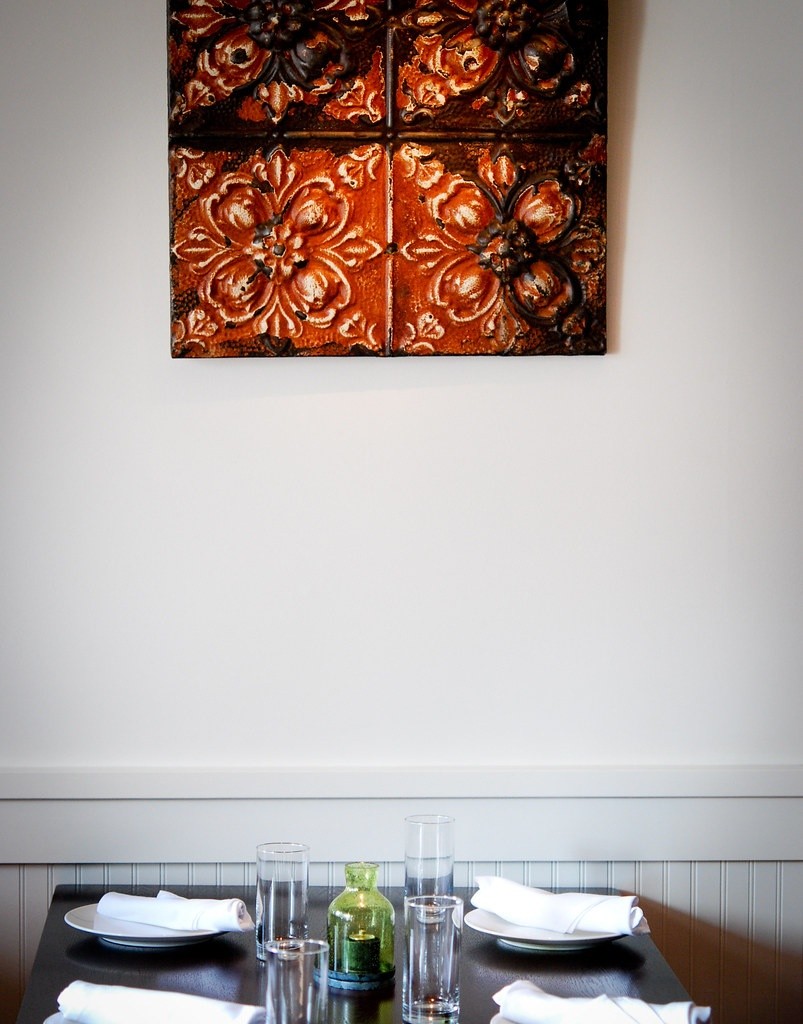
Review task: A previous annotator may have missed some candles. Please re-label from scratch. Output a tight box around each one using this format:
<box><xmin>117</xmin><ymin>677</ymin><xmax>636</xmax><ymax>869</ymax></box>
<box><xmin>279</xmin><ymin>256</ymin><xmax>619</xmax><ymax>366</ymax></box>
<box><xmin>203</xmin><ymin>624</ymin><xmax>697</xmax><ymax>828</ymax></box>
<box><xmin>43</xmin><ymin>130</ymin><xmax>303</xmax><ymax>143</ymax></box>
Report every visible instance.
<box><xmin>341</xmin><ymin>928</ymin><xmax>380</xmax><ymax>975</ymax></box>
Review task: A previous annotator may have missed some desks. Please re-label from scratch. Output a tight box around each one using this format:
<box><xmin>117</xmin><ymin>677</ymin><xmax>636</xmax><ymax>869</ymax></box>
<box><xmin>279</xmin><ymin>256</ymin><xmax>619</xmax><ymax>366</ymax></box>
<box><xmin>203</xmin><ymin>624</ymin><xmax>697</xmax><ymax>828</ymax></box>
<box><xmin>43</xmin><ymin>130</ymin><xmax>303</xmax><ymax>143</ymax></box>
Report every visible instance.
<box><xmin>21</xmin><ymin>885</ymin><xmax>696</xmax><ymax>1024</ymax></box>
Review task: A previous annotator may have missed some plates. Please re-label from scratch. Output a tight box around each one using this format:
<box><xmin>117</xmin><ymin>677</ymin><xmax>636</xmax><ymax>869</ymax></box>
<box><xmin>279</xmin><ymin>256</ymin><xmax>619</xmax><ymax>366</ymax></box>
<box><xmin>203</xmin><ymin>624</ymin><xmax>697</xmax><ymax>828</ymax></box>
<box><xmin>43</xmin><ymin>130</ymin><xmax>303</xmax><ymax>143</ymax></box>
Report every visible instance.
<box><xmin>65</xmin><ymin>904</ymin><xmax>230</xmax><ymax>952</ymax></box>
<box><xmin>464</xmin><ymin>907</ymin><xmax>628</xmax><ymax>952</ymax></box>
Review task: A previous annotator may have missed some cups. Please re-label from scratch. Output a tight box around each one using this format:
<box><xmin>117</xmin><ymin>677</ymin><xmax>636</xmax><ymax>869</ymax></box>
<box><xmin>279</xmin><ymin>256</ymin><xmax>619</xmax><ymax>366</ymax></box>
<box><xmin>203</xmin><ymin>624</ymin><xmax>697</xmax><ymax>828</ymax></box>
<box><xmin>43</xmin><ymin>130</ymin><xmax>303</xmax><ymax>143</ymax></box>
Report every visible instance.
<box><xmin>253</xmin><ymin>843</ymin><xmax>311</xmax><ymax>960</ymax></box>
<box><xmin>400</xmin><ymin>814</ymin><xmax>463</xmax><ymax>1024</ymax></box>
<box><xmin>264</xmin><ymin>942</ymin><xmax>330</xmax><ymax>1024</ymax></box>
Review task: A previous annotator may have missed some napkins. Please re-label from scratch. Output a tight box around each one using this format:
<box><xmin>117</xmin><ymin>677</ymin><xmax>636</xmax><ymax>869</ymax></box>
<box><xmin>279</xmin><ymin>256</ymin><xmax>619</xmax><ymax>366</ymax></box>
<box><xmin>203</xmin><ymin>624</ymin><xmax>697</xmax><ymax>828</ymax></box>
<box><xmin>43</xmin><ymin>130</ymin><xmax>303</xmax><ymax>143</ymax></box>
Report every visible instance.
<box><xmin>471</xmin><ymin>870</ymin><xmax>651</xmax><ymax>936</ymax></box>
<box><xmin>97</xmin><ymin>889</ymin><xmax>254</xmax><ymax>934</ymax></box>
<box><xmin>57</xmin><ymin>979</ymin><xmax>266</xmax><ymax>1024</ymax></box>
<box><xmin>492</xmin><ymin>979</ymin><xmax>711</xmax><ymax>1024</ymax></box>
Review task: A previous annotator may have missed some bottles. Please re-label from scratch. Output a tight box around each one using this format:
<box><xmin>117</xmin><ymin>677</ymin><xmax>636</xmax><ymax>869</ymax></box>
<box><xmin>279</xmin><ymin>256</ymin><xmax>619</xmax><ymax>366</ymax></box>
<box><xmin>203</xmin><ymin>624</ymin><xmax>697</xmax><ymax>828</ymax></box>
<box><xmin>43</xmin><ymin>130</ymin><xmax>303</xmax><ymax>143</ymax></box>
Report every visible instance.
<box><xmin>329</xmin><ymin>862</ymin><xmax>393</xmax><ymax>970</ymax></box>
